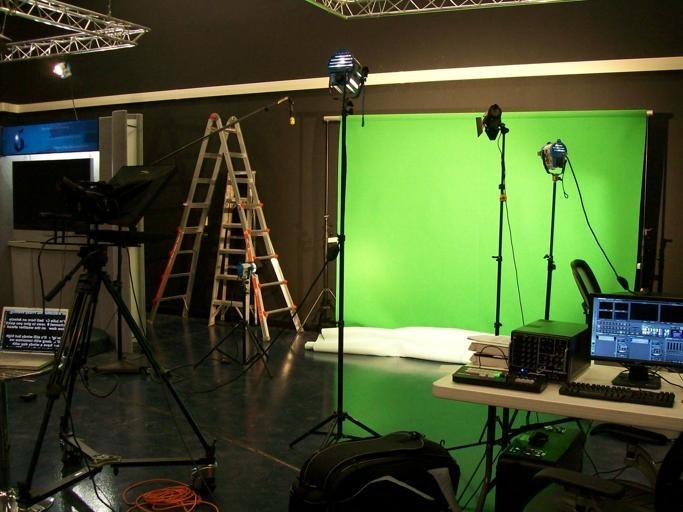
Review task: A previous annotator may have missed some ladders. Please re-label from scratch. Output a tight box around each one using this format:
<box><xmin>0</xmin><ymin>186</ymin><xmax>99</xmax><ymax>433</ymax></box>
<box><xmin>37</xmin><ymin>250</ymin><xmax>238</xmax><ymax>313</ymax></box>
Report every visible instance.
<box><xmin>147</xmin><ymin>113</ymin><xmax>305</xmax><ymax>334</ymax></box>
<box><xmin>209</xmin><ymin>170</ymin><xmax>258</xmax><ymax>326</ymax></box>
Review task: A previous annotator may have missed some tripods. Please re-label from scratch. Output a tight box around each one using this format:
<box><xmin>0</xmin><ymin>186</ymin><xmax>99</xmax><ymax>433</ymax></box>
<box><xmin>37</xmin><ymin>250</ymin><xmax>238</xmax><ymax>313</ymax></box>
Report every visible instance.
<box><xmin>193</xmin><ymin>285</ymin><xmax>274</xmax><ymax>380</ymax></box>
<box><xmin>22</xmin><ymin>244</ymin><xmax>219</xmax><ymax>505</ymax></box>
<box><xmin>287</xmin><ymin>113</ymin><xmax>383</xmax><ymax>461</ymax></box>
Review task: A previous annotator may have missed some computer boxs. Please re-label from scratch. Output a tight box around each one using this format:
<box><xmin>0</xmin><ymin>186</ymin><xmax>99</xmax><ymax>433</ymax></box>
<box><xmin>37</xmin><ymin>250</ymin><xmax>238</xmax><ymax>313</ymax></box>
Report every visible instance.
<box><xmin>493</xmin><ymin>424</ymin><xmax>584</xmax><ymax>512</ymax></box>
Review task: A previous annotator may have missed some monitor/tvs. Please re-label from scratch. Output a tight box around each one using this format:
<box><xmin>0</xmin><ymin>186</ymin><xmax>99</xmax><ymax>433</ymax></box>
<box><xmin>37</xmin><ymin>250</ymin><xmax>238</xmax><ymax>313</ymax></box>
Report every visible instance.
<box><xmin>12</xmin><ymin>159</ymin><xmax>92</xmax><ymax>232</ymax></box>
<box><xmin>588</xmin><ymin>294</ymin><xmax>683</xmax><ymax>389</ymax></box>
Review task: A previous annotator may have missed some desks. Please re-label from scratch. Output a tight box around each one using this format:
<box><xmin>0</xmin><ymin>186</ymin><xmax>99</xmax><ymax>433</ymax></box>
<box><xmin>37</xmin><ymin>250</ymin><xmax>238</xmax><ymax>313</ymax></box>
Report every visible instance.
<box><xmin>431</xmin><ymin>359</ymin><xmax>683</xmax><ymax>512</ymax></box>
<box><xmin>1</xmin><ymin>364</ymin><xmax>69</xmax><ymax>471</ymax></box>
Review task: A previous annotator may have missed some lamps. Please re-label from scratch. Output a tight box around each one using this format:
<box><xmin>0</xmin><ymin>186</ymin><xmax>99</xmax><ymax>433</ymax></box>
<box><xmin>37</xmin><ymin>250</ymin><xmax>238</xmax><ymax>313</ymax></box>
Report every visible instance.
<box><xmin>506</xmin><ymin>137</ymin><xmax>584</xmax><ymax>441</ymax></box>
<box><xmin>287</xmin><ymin>47</ymin><xmax>382</xmax><ymax>452</ymax></box>
<box><xmin>475</xmin><ymin>103</ymin><xmax>524</xmax><ymax>443</ymax></box>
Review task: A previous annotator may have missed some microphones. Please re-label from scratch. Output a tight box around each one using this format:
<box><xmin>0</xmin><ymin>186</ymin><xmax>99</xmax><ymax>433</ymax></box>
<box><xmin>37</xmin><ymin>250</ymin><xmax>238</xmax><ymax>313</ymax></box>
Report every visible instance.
<box><xmin>289</xmin><ymin>97</ymin><xmax>296</xmax><ymax>128</ymax></box>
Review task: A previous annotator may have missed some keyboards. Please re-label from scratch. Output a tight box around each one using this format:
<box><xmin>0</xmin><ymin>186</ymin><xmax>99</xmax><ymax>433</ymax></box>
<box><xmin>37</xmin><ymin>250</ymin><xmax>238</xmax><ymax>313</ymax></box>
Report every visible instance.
<box><xmin>558</xmin><ymin>382</ymin><xmax>677</xmax><ymax>408</ymax></box>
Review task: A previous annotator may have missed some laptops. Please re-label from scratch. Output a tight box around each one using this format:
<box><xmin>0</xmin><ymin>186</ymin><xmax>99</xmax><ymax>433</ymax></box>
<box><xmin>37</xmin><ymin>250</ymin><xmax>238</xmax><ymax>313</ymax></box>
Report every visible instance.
<box><xmin>0</xmin><ymin>306</ymin><xmax>69</xmax><ymax>370</ymax></box>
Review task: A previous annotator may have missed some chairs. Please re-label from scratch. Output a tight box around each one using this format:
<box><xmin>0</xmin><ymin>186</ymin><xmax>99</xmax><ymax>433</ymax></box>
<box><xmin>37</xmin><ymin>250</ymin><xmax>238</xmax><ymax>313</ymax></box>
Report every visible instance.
<box><xmin>524</xmin><ymin>433</ymin><xmax>682</xmax><ymax>512</ymax></box>
<box><xmin>570</xmin><ymin>257</ymin><xmax>604</xmax><ymax>327</ymax></box>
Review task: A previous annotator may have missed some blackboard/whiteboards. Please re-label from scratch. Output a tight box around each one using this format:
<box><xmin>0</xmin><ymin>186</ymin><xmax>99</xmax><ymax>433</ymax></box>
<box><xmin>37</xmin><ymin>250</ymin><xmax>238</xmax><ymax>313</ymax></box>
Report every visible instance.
<box><xmin>0</xmin><ymin>306</ymin><xmax>69</xmax><ymax>353</ymax></box>
